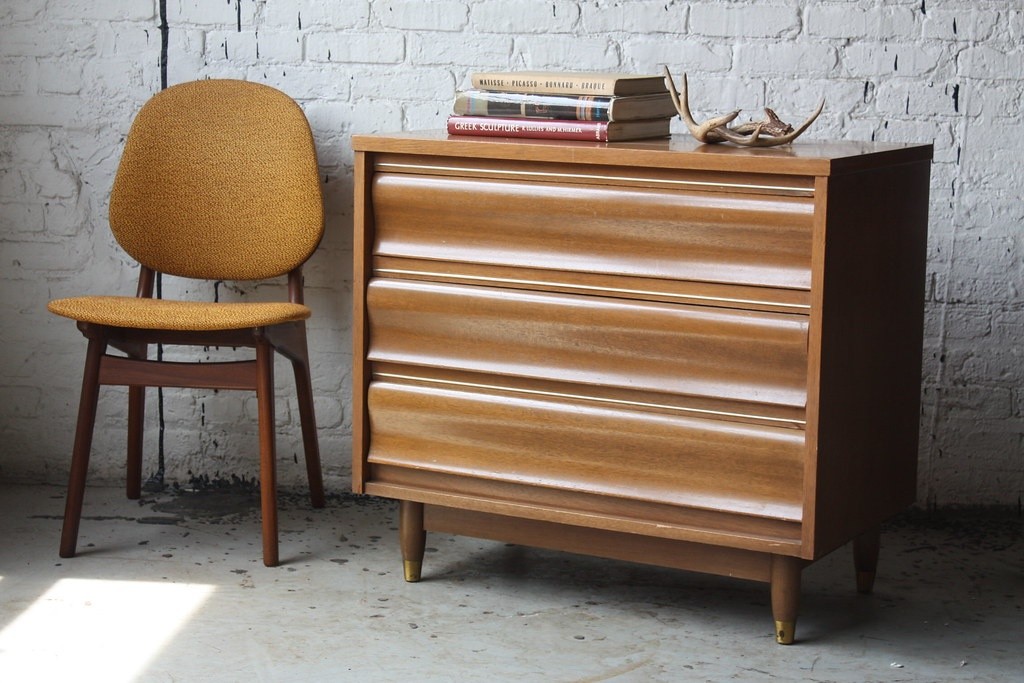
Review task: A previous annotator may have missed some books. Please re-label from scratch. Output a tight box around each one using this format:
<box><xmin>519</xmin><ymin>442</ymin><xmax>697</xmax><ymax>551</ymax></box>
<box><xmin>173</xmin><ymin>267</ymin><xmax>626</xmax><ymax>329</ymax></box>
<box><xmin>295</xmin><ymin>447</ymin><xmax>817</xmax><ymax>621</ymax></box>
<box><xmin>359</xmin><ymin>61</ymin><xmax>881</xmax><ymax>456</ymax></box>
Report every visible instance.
<box><xmin>447</xmin><ymin>72</ymin><xmax>681</xmax><ymax>142</ymax></box>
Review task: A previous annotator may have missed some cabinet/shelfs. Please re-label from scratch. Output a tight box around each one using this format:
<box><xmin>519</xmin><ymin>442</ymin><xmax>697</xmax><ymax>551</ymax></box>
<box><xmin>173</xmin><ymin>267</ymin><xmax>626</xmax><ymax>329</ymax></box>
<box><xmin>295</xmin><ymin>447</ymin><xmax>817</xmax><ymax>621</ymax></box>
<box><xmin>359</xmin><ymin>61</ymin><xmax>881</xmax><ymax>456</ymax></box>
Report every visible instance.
<box><xmin>352</xmin><ymin>131</ymin><xmax>933</xmax><ymax>644</ymax></box>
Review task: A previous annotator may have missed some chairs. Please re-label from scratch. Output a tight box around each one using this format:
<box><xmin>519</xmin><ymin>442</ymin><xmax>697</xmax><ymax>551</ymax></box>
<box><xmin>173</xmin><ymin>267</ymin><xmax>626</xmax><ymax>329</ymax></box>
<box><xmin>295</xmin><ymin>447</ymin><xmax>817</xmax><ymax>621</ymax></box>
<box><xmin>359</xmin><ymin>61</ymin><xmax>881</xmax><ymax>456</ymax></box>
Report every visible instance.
<box><xmin>48</xmin><ymin>79</ymin><xmax>325</xmax><ymax>567</ymax></box>
